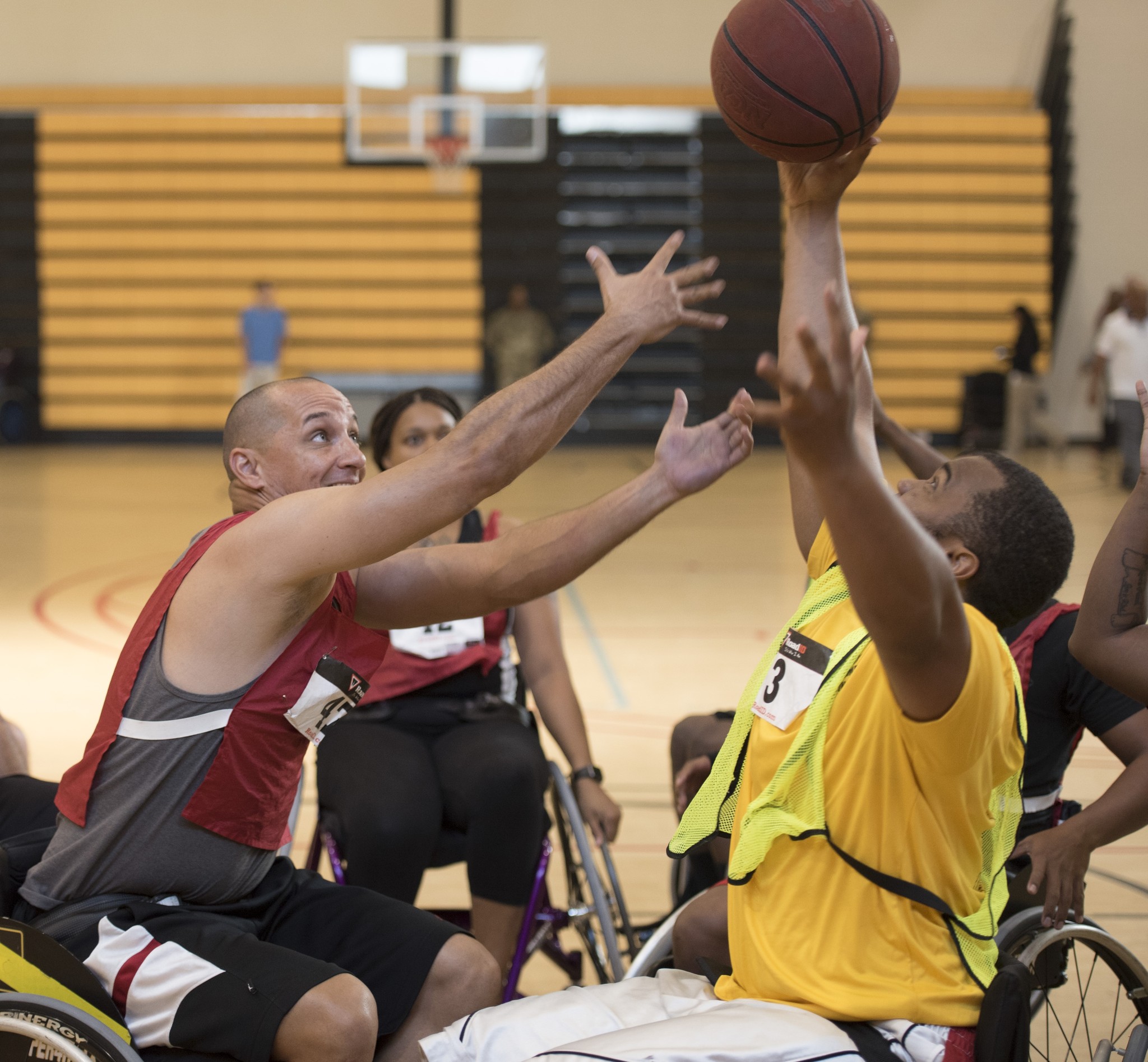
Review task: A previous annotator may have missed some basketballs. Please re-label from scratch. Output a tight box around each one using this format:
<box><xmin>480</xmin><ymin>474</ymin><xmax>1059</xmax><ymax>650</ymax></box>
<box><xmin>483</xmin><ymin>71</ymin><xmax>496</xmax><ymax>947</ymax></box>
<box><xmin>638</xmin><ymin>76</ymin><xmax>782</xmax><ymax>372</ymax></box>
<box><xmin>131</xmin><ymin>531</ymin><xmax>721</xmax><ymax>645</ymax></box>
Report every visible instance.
<box><xmin>710</xmin><ymin>1</ymin><xmax>905</xmax><ymax>166</ymax></box>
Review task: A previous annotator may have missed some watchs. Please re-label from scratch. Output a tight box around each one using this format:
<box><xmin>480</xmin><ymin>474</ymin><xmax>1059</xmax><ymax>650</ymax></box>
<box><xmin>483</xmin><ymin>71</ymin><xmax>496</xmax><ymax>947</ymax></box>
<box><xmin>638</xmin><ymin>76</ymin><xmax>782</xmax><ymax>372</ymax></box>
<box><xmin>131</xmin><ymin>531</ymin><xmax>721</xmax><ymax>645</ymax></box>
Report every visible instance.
<box><xmin>571</xmin><ymin>767</ymin><xmax>602</xmax><ymax>783</ymax></box>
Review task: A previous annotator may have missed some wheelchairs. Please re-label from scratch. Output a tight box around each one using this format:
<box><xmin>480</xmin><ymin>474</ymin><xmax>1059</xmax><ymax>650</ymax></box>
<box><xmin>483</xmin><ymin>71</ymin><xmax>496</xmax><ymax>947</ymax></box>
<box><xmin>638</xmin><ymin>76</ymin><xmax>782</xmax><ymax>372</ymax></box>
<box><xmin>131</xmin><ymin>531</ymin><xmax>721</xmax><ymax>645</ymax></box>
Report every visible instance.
<box><xmin>1</xmin><ymin>663</ymin><xmax>1147</xmax><ymax>1062</ymax></box>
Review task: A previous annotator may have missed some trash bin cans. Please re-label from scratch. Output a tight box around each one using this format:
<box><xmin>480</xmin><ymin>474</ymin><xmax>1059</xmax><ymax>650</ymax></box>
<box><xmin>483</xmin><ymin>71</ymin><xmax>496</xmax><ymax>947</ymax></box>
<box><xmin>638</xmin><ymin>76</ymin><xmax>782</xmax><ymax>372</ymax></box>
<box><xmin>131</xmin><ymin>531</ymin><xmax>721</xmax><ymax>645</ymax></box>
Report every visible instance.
<box><xmin>958</xmin><ymin>372</ymin><xmax>1004</xmax><ymax>450</ymax></box>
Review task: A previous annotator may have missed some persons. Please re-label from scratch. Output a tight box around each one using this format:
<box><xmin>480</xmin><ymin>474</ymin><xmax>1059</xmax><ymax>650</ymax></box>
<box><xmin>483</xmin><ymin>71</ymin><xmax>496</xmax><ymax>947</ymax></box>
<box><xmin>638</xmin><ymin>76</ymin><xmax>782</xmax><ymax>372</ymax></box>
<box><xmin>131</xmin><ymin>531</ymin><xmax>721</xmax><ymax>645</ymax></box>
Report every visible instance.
<box><xmin>485</xmin><ymin>281</ymin><xmax>556</xmax><ymax>390</ymax></box>
<box><xmin>318</xmin><ymin>387</ymin><xmax>621</xmax><ymax>1008</ymax></box>
<box><xmin>18</xmin><ymin>228</ymin><xmax>754</xmax><ymax>1062</ymax></box>
<box><xmin>238</xmin><ymin>280</ymin><xmax>287</xmax><ymax>397</ymax></box>
<box><xmin>672</xmin><ymin>137</ymin><xmax>1148</xmax><ymax>1028</ymax></box>
